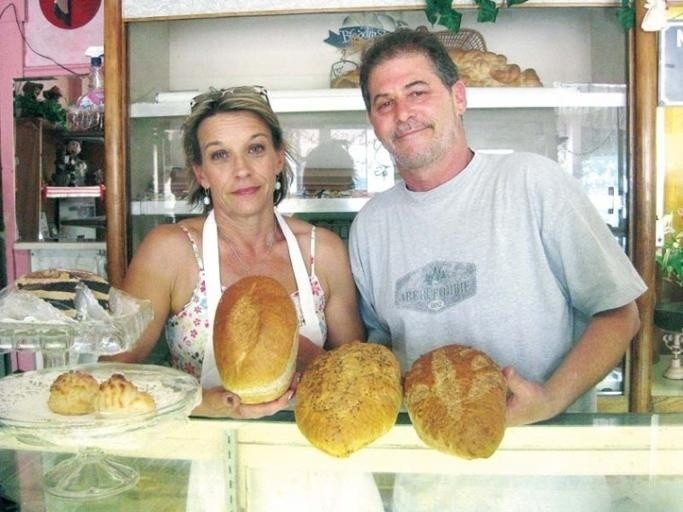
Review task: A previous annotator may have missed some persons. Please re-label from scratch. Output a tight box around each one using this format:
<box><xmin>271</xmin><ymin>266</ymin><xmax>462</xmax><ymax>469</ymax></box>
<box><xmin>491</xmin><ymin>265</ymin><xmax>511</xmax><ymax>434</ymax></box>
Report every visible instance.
<box><xmin>97</xmin><ymin>85</ymin><xmax>368</xmax><ymax>421</ymax></box>
<box><xmin>347</xmin><ymin>30</ymin><xmax>649</xmax><ymax>430</ymax></box>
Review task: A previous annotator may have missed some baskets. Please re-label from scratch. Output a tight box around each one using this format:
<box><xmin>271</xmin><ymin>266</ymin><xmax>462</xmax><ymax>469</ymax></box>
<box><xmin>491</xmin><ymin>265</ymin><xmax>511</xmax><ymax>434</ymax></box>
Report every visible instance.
<box><xmin>432</xmin><ymin>29</ymin><xmax>486</xmax><ymax>57</ymax></box>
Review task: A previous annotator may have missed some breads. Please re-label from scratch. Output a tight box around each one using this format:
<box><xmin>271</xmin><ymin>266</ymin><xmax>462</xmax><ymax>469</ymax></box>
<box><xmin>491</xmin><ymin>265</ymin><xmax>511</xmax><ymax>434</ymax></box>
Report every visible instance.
<box><xmin>48</xmin><ymin>369</ymin><xmax>156</xmax><ymax>417</ymax></box>
<box><xmin>330</xmin><ymin>47</ymin><xmax>544</xmax><ymax>87</ymax></box>
<box><xmin>214</xmin><ymin>275</ymin><xmax>299</xmax><ymax>405</ymax></box>
<box><xmin>294</xmin><ymin>340</ymin><xmax>404</xmax><ymax>458</ymax></box>
<box><xmin>404</xmin><ymin>343</ymin><xmax>507</xmax><ymax>460</ymax></box>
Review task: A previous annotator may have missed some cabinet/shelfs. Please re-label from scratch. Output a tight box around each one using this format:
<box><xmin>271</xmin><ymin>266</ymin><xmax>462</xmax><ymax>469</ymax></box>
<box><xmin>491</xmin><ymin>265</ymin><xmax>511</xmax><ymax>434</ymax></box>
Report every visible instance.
<box><xmin>11</xmin><ymin>118</ymin><xmax>110</xmax><ymax>285</ymax></box>
<box><xmin>102</xmin><ymin>1</ymin><xmax>657</xmax><ymax>415</ymax></box>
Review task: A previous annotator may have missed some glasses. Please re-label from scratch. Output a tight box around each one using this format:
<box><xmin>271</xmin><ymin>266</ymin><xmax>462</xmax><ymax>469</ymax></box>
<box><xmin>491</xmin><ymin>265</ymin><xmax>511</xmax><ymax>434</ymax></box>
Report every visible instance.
<box><xmin>189</xmin><ymin>85</ymin><xmax>273</xmax><ymax>116</ymax></box>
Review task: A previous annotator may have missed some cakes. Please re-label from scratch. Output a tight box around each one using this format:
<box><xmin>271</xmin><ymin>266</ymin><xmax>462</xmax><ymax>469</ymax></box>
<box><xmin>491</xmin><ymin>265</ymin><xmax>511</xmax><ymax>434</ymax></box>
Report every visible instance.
<box><xmin>15</xmin><ymin>268</ymin><xmax>110</xmax><ymax>320</ymax></box>
